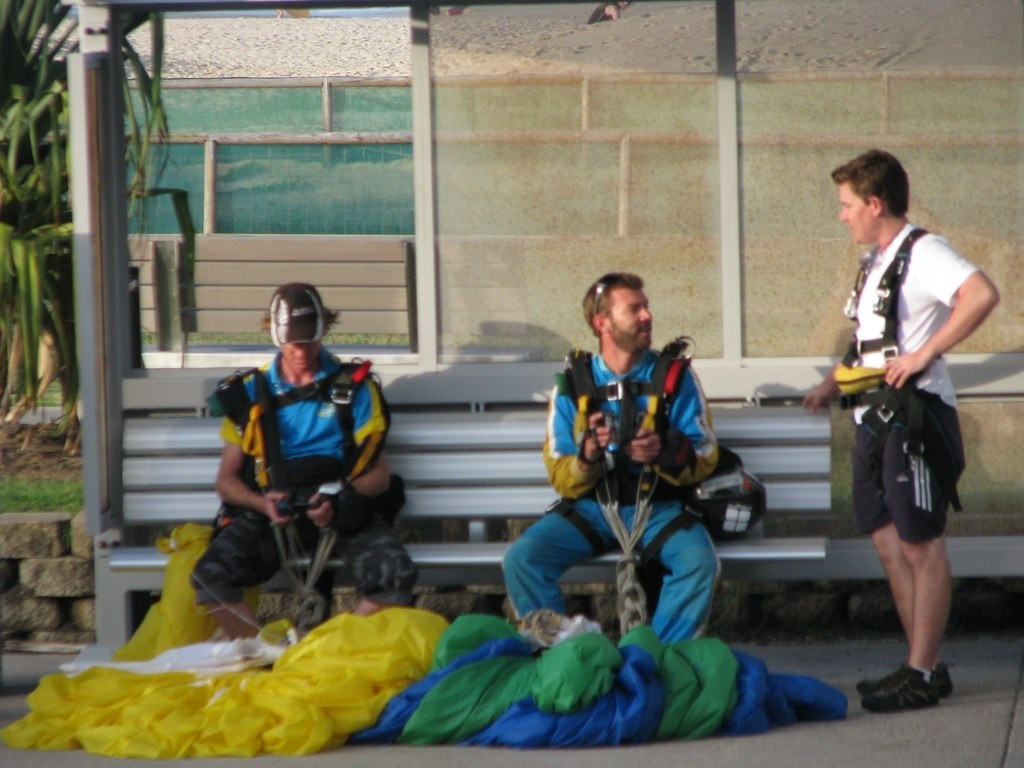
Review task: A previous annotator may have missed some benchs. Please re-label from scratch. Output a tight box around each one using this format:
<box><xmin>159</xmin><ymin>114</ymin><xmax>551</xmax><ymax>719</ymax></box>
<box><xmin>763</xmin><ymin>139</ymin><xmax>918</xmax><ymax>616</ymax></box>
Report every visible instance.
<box><xmin>108</xmin><ymin>411</ymin><xmax>831</xmax><ymax>646</ymax></box>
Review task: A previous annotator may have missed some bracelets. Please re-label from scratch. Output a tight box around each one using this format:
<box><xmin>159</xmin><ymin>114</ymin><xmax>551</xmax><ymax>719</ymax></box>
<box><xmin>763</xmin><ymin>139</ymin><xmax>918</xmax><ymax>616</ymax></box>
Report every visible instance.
<box><xmin>580</xmin><ymin>441</ymin><xmax>600</xmax><ymax>464</ymax></box>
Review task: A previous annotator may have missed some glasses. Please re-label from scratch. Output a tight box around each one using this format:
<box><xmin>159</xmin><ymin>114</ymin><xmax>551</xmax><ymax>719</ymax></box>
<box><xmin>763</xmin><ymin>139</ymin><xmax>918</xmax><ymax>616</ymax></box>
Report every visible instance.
<box><xmin>591</xmin><ymin>273</ymin><xmax>621</xmax><ymax>313</ymax></box>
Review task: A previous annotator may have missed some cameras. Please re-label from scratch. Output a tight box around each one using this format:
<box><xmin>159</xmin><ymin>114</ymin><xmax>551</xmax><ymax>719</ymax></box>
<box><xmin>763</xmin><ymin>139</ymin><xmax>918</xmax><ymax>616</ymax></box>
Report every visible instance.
<box><xmin>278</xmin><ymin>501</ymin><xmax>318</xmax><ymax>518</ymax></box>
<box><xmin>603</xmin><ymin>415</ymin><xmax>620</xmax><ymax>453</ymax></box>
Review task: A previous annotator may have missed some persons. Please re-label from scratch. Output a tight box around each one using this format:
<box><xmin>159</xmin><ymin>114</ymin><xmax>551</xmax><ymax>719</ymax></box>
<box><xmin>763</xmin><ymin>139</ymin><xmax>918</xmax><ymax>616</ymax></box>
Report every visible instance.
<box><xmin>502</xmin><ymin>273</ymin><xmax>720</xmax><ymax>645</ymax></box>
<box><xmin>802</xmin><ymin>149</ymin><xmax>1001</xmax><ymax>713</ymax></box>
<box><xmin>190</xmin><ymin>283</ymin><xmax>417</xmax><ymax>645</ymax></box>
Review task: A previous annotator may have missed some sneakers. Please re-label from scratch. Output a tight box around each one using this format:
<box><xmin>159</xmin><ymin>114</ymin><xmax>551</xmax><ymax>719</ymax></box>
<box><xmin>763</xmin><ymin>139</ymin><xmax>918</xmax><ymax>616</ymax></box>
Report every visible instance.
<box><xmin>861</xmin><ymin>665</ymin><xmax>942</xmax><ymax>713</ymax></box>
<box><xmin>855</xmin><ymin>664</ymin><xmax>954</xmax><ymax>700</ymax></box>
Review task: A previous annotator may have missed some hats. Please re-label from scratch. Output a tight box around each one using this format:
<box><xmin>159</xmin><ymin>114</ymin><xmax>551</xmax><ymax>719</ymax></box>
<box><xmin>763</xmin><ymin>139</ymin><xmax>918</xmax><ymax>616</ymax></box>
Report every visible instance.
<box><xmin>268</xmin><ymin>281</ymin><xmax>325</xmax><ymax>348</ymax></box>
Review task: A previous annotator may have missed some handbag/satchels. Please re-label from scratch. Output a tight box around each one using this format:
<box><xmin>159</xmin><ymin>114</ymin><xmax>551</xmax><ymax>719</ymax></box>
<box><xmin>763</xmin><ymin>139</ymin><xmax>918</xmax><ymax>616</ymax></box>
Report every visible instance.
<box><xmin>832</xmin><ymin>363</ymin><xmax>883</xmax><ymax>394</ymax></box>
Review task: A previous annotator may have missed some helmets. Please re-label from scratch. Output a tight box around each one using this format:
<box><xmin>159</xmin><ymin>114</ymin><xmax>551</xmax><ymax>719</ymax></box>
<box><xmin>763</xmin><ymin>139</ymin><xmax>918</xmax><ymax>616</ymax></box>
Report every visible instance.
<box><xmin>691</xmin><ymin>444</ymin><xmax>766</xmax><ymax>537</ymax></box>
